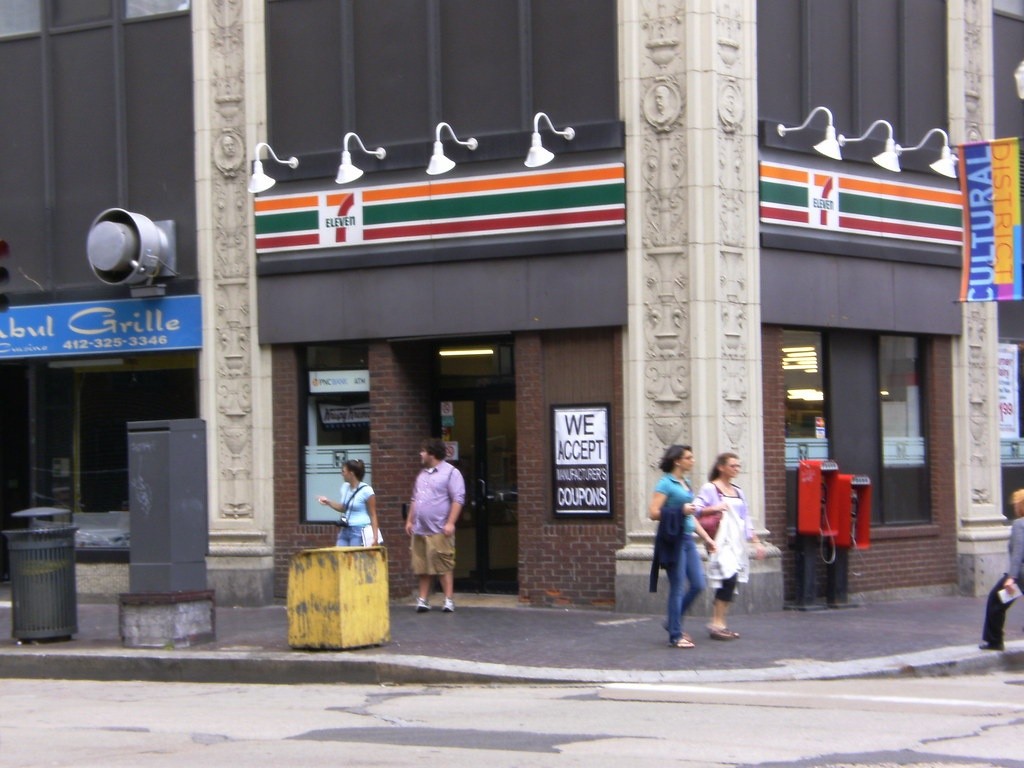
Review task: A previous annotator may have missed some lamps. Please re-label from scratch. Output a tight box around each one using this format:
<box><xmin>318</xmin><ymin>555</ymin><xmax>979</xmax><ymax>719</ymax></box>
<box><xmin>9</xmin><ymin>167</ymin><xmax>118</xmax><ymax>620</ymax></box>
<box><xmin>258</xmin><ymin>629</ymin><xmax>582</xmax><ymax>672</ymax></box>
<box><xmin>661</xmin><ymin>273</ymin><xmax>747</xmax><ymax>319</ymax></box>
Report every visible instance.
<box><xmin>837</xmin><ymin>120</ymin><xmax>903</xmax><ymax>173</ymax></box>
<box><xmin>247</xmin><ymin>143</ymin><xmax>299</xmax><ymax>193</ymax></box>
<box><xmin>776</xmin><ymin>106</ymin><xmax>842</xmax><ymax>161</ymax></box>
<box><xmin>524</xmin><ymin>112</ymin><xmax>575</xmax><ymax>167</ymax></box>
<box><xmin>894</xmin><ymin>129</ymin><xmax>959</xmax><ymax>178</ymax></box>
<box><xmin>335</xmin><ymin>132</ymin><xmax>386</xmax><ymax>184</ymax></box>
<box><xmin>426</xmin><ymin>122</ymin><xmax>478</xmax><ymax>175</ymax></box>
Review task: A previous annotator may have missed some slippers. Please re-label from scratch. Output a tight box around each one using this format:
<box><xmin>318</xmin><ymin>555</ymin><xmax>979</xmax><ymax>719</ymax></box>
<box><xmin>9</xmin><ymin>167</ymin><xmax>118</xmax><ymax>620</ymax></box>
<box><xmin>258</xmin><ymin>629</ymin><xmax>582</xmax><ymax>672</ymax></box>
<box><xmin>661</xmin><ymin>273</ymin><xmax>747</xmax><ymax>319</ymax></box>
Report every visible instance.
<box><xmin>668</xmin><ymin>640</ymin><xmax>694</xmax><ymax>649</ymax></box>
<box><xmin>665</xmin><ymin>624</ymin><xmax>692</xmax><ymax>642</ymax></box>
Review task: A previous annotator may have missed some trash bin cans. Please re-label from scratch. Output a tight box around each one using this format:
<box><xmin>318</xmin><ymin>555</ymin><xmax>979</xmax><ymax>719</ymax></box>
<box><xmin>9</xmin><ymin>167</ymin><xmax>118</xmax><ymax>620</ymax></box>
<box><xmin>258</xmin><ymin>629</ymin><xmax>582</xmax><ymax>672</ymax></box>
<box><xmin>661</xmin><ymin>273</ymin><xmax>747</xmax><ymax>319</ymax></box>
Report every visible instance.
<box><xmin>2</xmin><ymin>505</ymin><xmax>80</xmax><ymax>645</ymax></box>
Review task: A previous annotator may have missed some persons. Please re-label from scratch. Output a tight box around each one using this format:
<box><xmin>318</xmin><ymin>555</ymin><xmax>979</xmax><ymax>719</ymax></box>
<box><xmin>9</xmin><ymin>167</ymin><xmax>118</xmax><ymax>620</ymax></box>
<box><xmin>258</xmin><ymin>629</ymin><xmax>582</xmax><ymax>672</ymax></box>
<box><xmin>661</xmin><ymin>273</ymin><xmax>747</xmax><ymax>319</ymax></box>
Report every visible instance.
<box><xmin>649</xmin><ymin>444</ymin><xmax>715</xmax><ymax>649</ymax></box>
<box><xmin>980</xmin><ymin>489</ymin><xmax>1024</xmax><ymax>656</ymax></box>
<box><xmin>691</xmin><ymin>453</ymin><xmax>759</xmax><ymax>641</ymax></box>
<box><xmin>405</xmin><ymin>438</ymin><xmax>466</xmax><ymax>612</ymax></box>
<box><xmin>315</xmin><ymin>460</ymin><xmax>379</xmax><ymax>548</ymax></box>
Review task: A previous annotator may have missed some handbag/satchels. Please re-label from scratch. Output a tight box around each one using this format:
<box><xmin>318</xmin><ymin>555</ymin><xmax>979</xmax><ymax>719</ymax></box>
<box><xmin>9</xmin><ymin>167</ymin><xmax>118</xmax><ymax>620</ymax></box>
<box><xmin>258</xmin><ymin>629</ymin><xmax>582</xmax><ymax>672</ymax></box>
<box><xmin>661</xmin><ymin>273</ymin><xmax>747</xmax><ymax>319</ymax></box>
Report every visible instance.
<box><xmin>361</xmin><ymin>525</ymin><xmax>384</xmax><ymax>548</ymax></box>
<box><xmin>695</xmin><ymin>482</ymin><xmax>722</xmax><ymax>540</ymax></box>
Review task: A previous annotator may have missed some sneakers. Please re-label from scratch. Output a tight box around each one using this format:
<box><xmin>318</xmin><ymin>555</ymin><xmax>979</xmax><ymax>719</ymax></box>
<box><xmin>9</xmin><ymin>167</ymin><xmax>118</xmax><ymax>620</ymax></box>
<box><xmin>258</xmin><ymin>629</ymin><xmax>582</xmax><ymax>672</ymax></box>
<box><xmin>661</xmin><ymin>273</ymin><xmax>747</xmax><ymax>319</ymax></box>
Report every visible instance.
<box><xmin>415</xmin><ymin>596</ymin><xmax>432</xmax><ymax>611</ymax></box>
<box><xmin>442</xmin><ymin>597</ymin><xmax>455</xmax><ymax>612</ymax></box>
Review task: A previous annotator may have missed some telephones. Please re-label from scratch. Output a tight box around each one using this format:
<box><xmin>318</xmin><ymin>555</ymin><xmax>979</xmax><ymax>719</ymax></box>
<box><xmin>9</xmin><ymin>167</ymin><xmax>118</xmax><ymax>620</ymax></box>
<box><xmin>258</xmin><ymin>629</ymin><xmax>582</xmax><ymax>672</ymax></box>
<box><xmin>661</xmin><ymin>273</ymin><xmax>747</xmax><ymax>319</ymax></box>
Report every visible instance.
<box><xmin>851</xmin><ymin>489</ymin><xmax>858</xmax><ymax>522</ymax></box>
<box><xmin>821</xmin><ymin>476</ymin><xmax>827</xmax><ymax>506</ymax></box>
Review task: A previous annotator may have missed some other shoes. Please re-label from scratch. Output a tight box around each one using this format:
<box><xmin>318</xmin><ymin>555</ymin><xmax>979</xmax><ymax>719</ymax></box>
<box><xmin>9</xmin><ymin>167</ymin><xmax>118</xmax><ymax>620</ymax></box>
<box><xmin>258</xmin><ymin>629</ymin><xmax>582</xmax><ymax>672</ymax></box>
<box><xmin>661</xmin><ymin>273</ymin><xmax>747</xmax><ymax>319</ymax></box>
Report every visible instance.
<box><xmin>979</xmin><ymin>642</ymin><xmax>1004</xmax><ymax>651</ymax></box>
<box><xmin>710</xmin><ymin>626</ymin><xmax>740</xmax><ymax>640</ymax></box>
